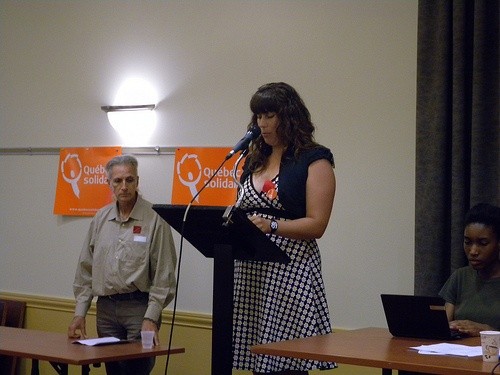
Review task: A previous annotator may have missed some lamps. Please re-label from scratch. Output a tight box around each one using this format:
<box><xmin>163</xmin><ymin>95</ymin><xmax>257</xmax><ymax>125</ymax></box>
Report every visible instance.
<box><xmin>101</xmin><ymin>104</ymin><xmax>155</xmax><ymax>113</ymax></box>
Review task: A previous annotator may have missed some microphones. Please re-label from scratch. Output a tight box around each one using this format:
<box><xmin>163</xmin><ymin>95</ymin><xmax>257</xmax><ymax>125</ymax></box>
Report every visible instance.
<box><xmin>225</xmin><ymin>125</ymin><xmax>261</xmax><ymax>161</ymax></box>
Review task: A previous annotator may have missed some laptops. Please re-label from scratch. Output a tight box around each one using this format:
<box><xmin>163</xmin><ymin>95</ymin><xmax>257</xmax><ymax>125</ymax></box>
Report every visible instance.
<box><xmin>381</xmin><ymin>293</ymin><xmax>468</xmax><ymax>341</ymax></box>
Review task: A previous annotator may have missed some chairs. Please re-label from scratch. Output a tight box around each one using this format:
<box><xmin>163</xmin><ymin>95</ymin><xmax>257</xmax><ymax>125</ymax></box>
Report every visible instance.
<box><xmin>0</xmin><ymin>298</ymin><xmax>26</xmax><ymax>375</ymax></box>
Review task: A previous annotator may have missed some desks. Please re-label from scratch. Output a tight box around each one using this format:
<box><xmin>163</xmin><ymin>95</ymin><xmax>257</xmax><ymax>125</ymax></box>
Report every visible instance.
<box><xmin>251</xmin><ymin>327</ymin><xmax>500</xmax><ymax>375</ymax></box>
<box><xmin>0</xmin><ymin>326</ymin><xmax>185</xmax><ymax>375</ymax></box>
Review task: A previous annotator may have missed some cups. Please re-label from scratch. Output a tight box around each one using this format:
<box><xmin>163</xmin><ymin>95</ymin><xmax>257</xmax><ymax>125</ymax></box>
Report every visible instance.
<box><xmin>141</xmin><ymin>331</ymin><xmax>155</xmax><ymax>350</ymax></box>
<box><xmin>480</xmin><ymin>330</ymin><xmax>500</xmax><ymax>363</ymax></box>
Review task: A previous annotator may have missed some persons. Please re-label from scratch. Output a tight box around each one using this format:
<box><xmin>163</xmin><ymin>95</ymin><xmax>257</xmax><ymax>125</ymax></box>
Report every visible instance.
<box><xmin>67</xmin><ymin>156</ymin><xmax>176</xmax><ymax>375</ymax></box>
<box><xmin>440</xmin><ymin>204</ymin><xmax>500</xmax><ymax>337</ymax></box>
<box><xmin>232</xmin><ymin>82</ymin><xmax>338</xmax><ymax>375</ymax></box>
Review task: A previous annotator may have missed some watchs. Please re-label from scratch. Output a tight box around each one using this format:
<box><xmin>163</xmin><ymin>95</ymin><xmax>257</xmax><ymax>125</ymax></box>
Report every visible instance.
<box><xmin>270</xmin><ymin>220</ymin><xmax>278</xmax><ymax>234</ymax></box>
